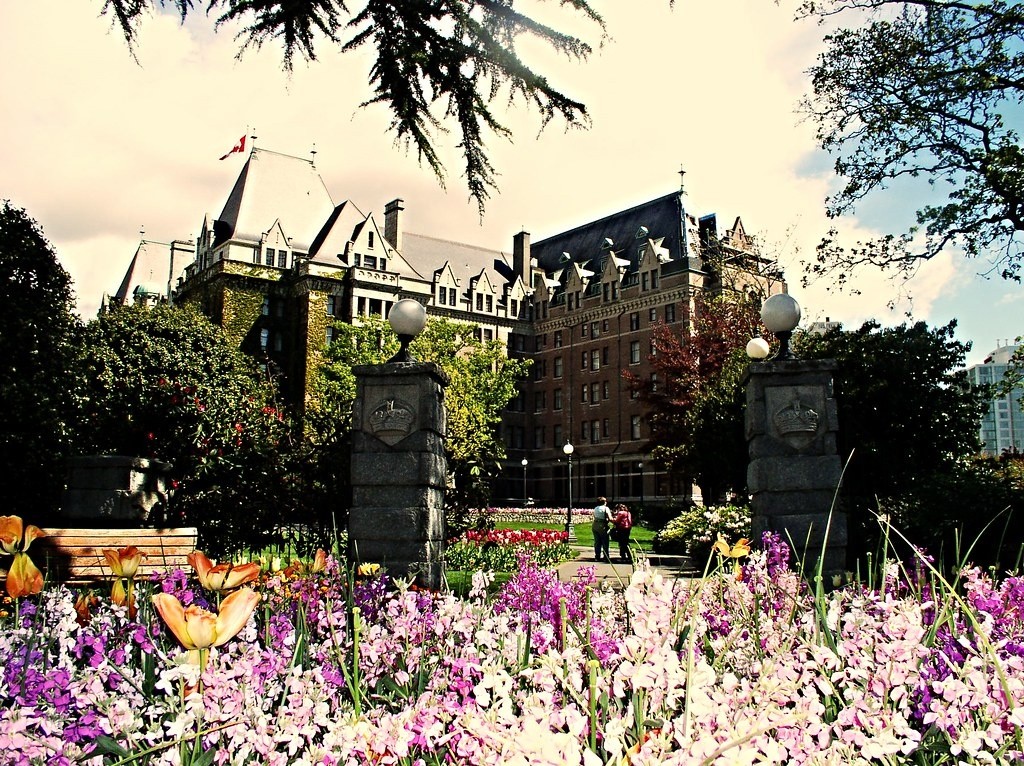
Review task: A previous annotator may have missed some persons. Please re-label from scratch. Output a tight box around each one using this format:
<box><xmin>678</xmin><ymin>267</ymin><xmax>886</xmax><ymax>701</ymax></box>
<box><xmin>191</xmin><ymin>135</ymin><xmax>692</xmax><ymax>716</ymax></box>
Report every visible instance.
<box><xmin>592</xmin><ymin>497</ymin><xmax>614</xmax><ymax>562</ymax></box>
<box><xmin>611</xmin><ymin>504</ymin><xmax>634</xmax><ymax>562</ymax></box>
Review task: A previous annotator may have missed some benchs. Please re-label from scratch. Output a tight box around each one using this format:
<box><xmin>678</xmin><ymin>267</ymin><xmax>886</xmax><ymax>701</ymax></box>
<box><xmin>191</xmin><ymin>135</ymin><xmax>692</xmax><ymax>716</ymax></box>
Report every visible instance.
<box><xmin>40</xmin><ymin>528</ymin><xmax>201</xmax><ymax>589</ymax></box>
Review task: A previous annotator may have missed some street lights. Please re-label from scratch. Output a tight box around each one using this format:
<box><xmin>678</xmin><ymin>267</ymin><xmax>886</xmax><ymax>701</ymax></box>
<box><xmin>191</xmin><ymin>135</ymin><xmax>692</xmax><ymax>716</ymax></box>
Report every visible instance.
<box><xmin>521</xmin><ymin>457</ymin><xmax>528</xmax><ymax>508</ymax></box>
<box><xmin>638</xmin><ymin>460</ymin><xmax>644</xmax><ymax>513</ymax></box>
<box><xmin>562</xmin><ymin>439</ymin><xmax>578</xmax><ymax>543</ymax></box>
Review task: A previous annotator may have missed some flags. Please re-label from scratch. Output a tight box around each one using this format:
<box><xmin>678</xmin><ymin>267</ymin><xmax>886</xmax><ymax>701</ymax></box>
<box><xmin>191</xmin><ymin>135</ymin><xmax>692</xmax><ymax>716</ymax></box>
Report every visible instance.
<box><xmin>219</xmin><ymin>134</ymin><xmax>246</xmax><ymax>161</ymax></box>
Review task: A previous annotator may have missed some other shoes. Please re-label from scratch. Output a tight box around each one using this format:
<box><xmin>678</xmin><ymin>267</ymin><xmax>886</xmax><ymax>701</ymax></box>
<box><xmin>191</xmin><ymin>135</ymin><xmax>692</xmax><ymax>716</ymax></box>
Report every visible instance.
<box><xmin>617</xmin><ymin>558</ymin><xmax>626</xmax><ymax>562</ymax></box>
<box><xmin>626</xmin><ymin>559</ymin><xmax>633</xmax><ymax>564</ymax></box>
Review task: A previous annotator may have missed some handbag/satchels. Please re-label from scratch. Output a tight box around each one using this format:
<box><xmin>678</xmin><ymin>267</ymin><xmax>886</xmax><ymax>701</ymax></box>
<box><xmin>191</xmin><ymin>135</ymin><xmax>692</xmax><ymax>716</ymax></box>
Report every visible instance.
<box><xmin>609</xmin><ymin>527</ymin><xmax>622</xmax><ymax>542</ymax></box>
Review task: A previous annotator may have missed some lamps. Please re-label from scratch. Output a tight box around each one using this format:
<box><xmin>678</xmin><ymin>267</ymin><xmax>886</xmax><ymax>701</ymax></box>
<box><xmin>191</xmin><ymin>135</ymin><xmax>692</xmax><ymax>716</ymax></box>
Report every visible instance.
<box><xmin>746</xmin><ymin>338</ymin><xmax>769</xmax><ymax>363</ymax></box>
<box><xmin>760</xmin><ymin>294</ymin><xmax>804</xmax><ymax>362</ymax></box>
<box><xmin>388</xmin><ymin>298</ymin><xmax>428</xmax><ymax>362</ymax></box>
